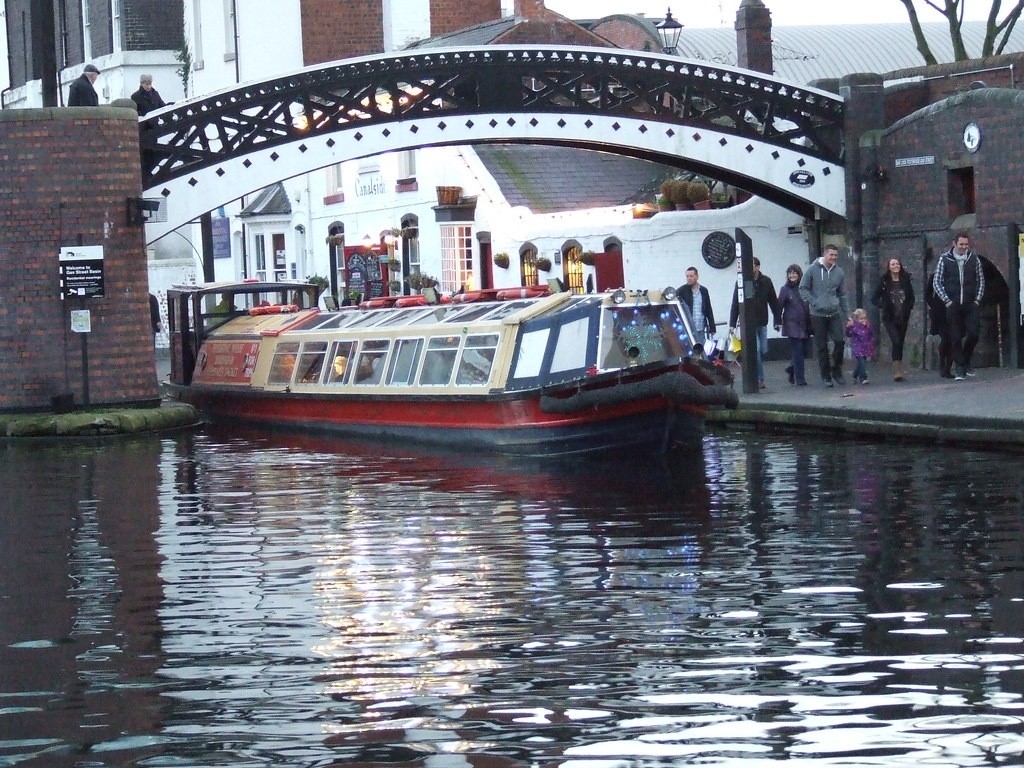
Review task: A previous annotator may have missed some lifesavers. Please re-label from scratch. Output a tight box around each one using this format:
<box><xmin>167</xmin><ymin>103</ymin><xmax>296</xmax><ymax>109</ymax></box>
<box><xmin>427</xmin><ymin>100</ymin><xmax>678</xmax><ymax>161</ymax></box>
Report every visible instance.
<box><xmin>249</xmin><ymin>304</ymin><xmax>298</xmax><ymax>315</ymax></box>
<box><xmin>454</xmin><ymin>292</ymin><xmax>496</xmax><ymax>303</ymax></box>
<box><xmin>243</xmin><ymin>279</ymin><xmax>258</xmax><ymax>282</ymax></box>
<box><xmin>395</xmin><ymin>296</ymin><xmax>451</xmax><ymax>308</ymax></box>
<box><xmin>496</xmin><ymin>288</ymin><xmax>551</xmax><ymax>301</ymax></box>
<box><xmin>360</xmin><ymin>299</ymin><xmax>396</xmax><ymax>308</ymax></box>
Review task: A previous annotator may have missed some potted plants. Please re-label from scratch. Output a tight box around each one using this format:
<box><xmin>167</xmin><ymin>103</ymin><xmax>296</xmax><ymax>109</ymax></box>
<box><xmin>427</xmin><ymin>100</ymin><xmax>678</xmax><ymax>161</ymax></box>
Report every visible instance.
<box><xmin>532</xmin><ymin>256</ymin><xmax>551</xmax><ymax>272</ymax></box>
<box><xmin>403</xmin><ymin>272</ymin><xmax>439</xmax><ymax>303</ymax></box>
<box><xmin>387</xmin><ymin>281</ymin><xmax>400</xmax><ymax>296</ymax></box>
<box><xmin>493</xmin><ymin>253</ymin><xmax>509</xmax><ymax>269</ymax></box>
<box><xmin>387</xmin><ymin>259</ymin><xmax>401</xmax><ymax>271</ymax></box>
<box><xmin>325</xmin><ymin>234</ymin><xmax>344</xmax><ymax>246</ymax></box>
<box><xmin>401</xmin><ymin>229</ymin><xmax>415</xmax><ymax>239</ymax></box>
<box><xmin>658</xmin><ymin>180</ymin><xmax>732</xmax><ymax>212</ymax></box>
<box><xmin>348</xmin><ymin>291</ymin><xmax>359</xmax><ymax>306</ymax></box>
<box><xmin>578</xmin><ymin>251</ymin><xmax>595</xmax><ymax>266</ymax></box>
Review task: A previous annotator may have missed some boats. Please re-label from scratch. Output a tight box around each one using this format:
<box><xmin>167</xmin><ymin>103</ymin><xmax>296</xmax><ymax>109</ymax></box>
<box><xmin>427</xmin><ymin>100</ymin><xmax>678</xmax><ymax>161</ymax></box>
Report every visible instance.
<box><xmin>161</xmin><ymin>278</ymin><xmax>740</xmax><ymax>458</ymax></box>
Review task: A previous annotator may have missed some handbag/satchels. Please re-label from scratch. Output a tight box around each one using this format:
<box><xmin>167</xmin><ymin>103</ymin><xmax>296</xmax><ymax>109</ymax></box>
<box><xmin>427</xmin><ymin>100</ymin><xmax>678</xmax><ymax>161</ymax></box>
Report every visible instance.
<box><xmin>727</xmin><ymin>333</ymin><xmax>742</xmax><ymax>352</ymax></box>
<box><xmin>805</xmin><ymin>313</ymin><xmax>815</xmax><ymax>336</ymax></box>
<box><xmin>703</xmin><ymin>335</ymin><xmax>716</xmax><ymax>356</ymax></box>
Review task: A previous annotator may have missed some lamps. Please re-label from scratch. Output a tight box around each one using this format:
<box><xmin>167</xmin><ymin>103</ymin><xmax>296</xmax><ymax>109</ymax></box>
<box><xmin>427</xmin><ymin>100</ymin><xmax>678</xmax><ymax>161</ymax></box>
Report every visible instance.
<box><xmin>131</xmin><ymin>198</ymin><xmax>160</xmax><ymax>226</ymax></box>
<box><xmin>295</xmin><ymin>226</ymin><xmax>304</xmax><ymax>234</ymax></box>
<box><xmin>654</xmin><ymin>5</ymin><xmax>684</xmax><ymax>55</ymax></box>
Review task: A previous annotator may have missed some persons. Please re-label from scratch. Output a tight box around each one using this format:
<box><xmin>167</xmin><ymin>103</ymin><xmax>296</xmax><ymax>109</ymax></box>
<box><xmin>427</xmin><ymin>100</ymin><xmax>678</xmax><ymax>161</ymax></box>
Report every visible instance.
<box><xmin>131</xmin><ymin>74</ymin><xmax>172</xmax><ymax>177</ymax></box>
<box><xmin>211</xmin><ymin>292</ymin><xmax>238</xmax><ymax>327</ymax></box>
<box><xmin>729</xmin><ymin>257</ymin><xmax>778</xmax><ymax>389</ymax></box>
<box><xmin>774</xmin><ymin>264</ymin><xmax>811</xmax><ymax>386</ymax></box>
<box><xmin>872</xmin><ymin>256</ymin><xmax>916</xmax><ymax>382</ymax></box>
<box><xmin>845</xmin><ymin>309</ymin><xmax>874</xmax><ymax>385</ymax></box>
<box><xmin>676</xmin><ymin>267</ymin><xmax>716</xmax><ymax>343</ymax></box>
<box><xmin>797</xmin><ymin>244</ymin><xmax>851</xmax><ymax>388</ymax></box>
<box><xmin>67</xmin><ymin>65</ymin><xmax>102</xmax><ymax>107</ymax></box>
<box><xmin>924</xmin><ymin>272</ymin><xmax>967</xmax><ymax>379</ymax></box>
<box><xmin>933</xmin><ymin>231</ymin><xmax>985</xmax><ymax>382</ymax></box>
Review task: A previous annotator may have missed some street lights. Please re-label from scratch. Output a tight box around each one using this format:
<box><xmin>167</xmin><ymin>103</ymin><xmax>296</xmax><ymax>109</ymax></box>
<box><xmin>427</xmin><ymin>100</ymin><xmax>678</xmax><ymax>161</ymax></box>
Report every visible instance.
<box><xmin>655</xmin><ymin>7</ymin><xmax>684</xmax><ymax>113</ymax></box>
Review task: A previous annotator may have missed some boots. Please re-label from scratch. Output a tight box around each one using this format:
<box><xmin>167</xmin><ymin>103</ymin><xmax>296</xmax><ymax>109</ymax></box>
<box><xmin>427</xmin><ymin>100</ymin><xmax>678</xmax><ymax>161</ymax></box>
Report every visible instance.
<box><xmin>892</xmin><ymin>360</ymin><xmax>905</xmax><ymax>382</ymax></box>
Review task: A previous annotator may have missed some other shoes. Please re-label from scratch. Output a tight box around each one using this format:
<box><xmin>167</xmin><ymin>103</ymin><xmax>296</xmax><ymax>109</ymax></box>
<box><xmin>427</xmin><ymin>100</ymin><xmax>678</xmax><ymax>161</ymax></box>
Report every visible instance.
<box><xmin>955</xmin><ymin>371</ymin><xmax>966</xmax><ymax>381</ymax></box>
<box><xmin>798</xmin><ymin>382</ymin><xmax>808</xmax><ymax>386</ymax></box>
<box><xmin>861</xmin><ymin>379</ymin><xmax>870</xmax><ymax>384</ymax></box>
<box><xmin>941</xmin><ymin>370</ymin><xmax>956</xmax><ymax>379</ymax></box>
<box><xmin>832</xmin><ymin>369</ymin><xmax>845</xmax><ymax>385</ymax></box>
<box><xmin>851</xmin><ymin>374</ymin><xmax>858</xmax><ymax>385</ymax></box>
<box><xmin>964</xmin><ymin>365</ymin><xmax>976</xmax><ymax>376</ymax></box>
<box><xmin>758</xmin><ymin>379</ymin><xmax>766</xmax><ymax>389</ymax></box>
<box><xmin>785</xmin><ymin>367</ymin><xmax>795</xmax><ymax>384</ymax></box>
<box><xmin>823</xmin><ymin>378</ymin><xmax>834</xmax><ymax>388</ymax></box>
